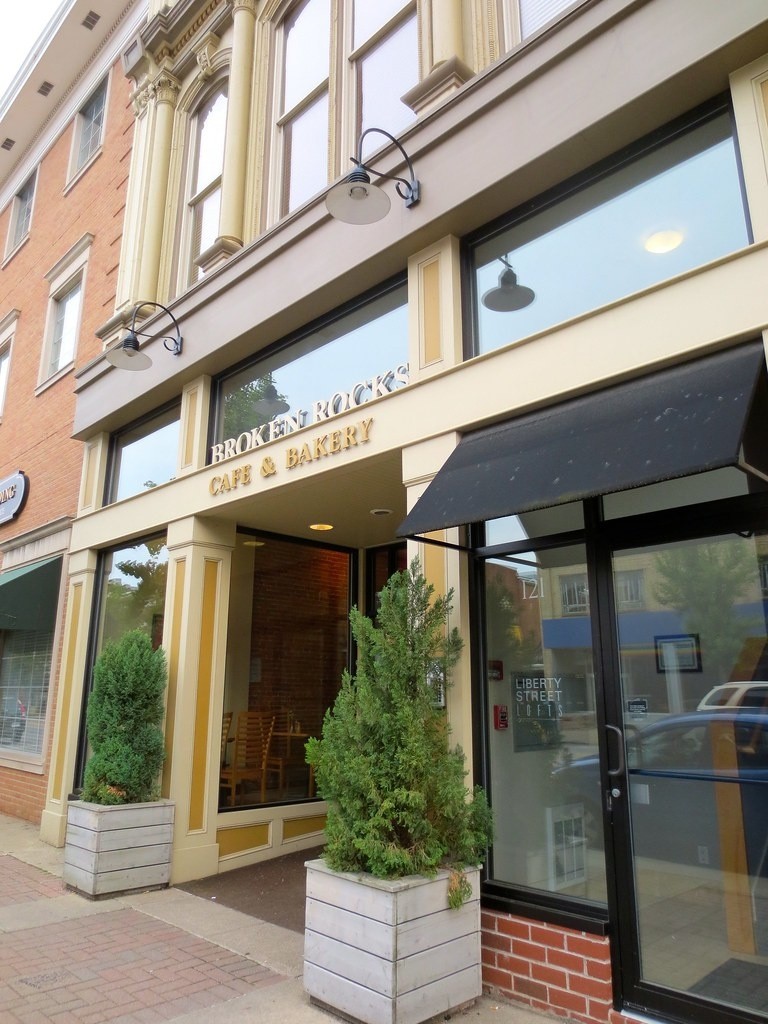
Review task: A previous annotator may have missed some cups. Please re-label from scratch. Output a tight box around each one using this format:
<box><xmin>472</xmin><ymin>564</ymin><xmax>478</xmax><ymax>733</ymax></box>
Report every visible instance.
<box><xmin>295</xmin><ymin>722</ymin><xmax>301</xmax><ymax>734</ymax></box>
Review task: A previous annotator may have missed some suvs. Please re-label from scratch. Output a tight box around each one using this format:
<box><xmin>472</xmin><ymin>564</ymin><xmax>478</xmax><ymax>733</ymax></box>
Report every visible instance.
<box><xmin>698</xmin><ymin>678</ymin><xmax>768</xmax><ymax>748</ymax></box>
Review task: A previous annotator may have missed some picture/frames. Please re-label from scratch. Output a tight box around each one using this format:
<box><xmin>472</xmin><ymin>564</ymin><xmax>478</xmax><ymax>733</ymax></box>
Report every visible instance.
<box><xmin>654</xmin><ymin>633</ymin><xmax>703</xmax><ymax>673</ymax></box>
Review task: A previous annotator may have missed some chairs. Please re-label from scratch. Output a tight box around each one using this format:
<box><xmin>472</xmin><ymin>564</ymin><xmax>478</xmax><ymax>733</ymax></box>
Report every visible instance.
<box><xmin>219</xmin><ymin>707</ymin><xmax>316</xmax><ymax>803</ymax></box>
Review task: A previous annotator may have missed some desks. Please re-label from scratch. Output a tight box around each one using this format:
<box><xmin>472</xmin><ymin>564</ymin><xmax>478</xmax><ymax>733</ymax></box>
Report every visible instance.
<box><xmin>271</xmin><ymin>731</ymin><xmax>313</xmax><ymax>798</ymax></box>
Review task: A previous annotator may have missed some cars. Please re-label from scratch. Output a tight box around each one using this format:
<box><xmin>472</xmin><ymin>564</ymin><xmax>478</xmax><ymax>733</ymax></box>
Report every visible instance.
<box><xmin>0</xmin><ymin>696</ymin><xmax>26</xmax><ymax>742</ymax></box>
<box><xmin>552</xmin><ymin>711</ymin><xmax>768</xmax><ymax>878</ymax></box>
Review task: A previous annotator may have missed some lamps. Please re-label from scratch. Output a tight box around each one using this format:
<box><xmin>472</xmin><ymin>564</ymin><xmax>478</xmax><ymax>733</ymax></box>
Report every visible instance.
<box><xmin>251</xmin><ymin>370</ymin><xmax>290</xmax><ymax>418</ymax></box>
<box><xmin>105</xmin><ymin>302</ymin><xmax>184</xmax><ymax>370</ymax></box>
<box><xmin>479</xmin><ymin>255</ymin><xmax>535</xmax><ymax>313</ymax></box>
<box><xmin>325</xmin><ymin>127</ymin><xmax>420</xmax><ymax>223</ymax></box>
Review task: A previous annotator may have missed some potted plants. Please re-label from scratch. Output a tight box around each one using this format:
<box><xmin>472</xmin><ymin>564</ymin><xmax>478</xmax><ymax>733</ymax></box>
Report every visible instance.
<box><xmin>63</xmin><ymin>624</ymin><xmax>177</xmax><ymax>900</ymax></box>
<box><xmin>297</xmin><ymin>558</ymin><xmax>492</xmax><ymax>1024</ymax></box>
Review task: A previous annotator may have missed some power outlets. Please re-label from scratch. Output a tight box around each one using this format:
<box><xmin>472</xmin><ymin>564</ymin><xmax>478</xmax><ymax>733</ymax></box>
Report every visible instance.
<box><xmin>697</xmin><ymin>846</ymin><xmax>710</xmax><ymax>864</ymax></box>
